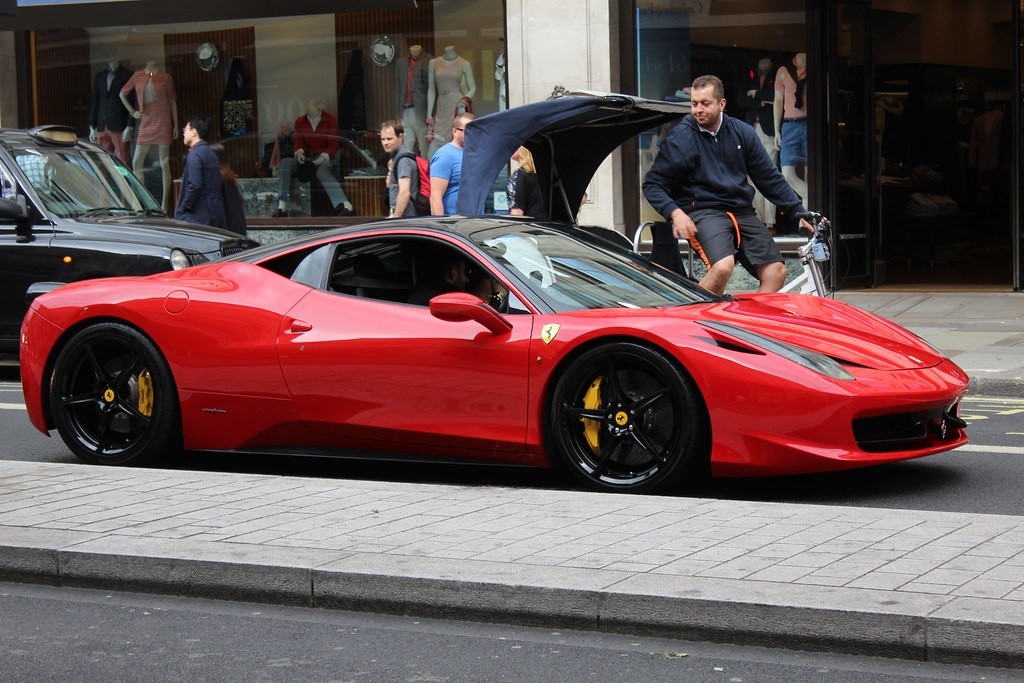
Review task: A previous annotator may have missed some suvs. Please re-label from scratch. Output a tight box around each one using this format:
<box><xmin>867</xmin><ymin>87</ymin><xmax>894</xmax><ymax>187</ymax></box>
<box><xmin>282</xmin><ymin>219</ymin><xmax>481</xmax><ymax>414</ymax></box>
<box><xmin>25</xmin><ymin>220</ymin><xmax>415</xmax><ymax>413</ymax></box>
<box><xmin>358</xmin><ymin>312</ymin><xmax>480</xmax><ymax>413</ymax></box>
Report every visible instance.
<box><xmin>0</xmin><ymin>124</ymin><xmax>262</xmax><ymax>366</ymax></box>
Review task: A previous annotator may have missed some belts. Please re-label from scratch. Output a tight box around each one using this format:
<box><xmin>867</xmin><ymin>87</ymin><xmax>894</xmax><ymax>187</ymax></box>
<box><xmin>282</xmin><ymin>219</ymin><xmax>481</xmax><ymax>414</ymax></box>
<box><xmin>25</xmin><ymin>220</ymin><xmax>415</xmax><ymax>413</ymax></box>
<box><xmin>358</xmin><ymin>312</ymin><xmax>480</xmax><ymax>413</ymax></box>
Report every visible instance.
<box><xmin>403</xmin><ymin>104</ymin><xmax>414</xmax><ymax>108</ymax></box>
<box><xmin>783</xmin><ymin>117</ymin><xmax>807</xmax><ymax>122</ymax></box>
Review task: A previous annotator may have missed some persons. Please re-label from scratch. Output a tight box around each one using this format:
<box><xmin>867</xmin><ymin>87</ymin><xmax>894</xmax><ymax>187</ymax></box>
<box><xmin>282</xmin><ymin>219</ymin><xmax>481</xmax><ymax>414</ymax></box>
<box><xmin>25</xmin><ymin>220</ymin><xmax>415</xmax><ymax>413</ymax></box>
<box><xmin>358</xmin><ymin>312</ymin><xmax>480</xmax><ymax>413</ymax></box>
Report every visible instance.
<box><xmin>379</xmin><ymin>119</ymin><xmax>419</xmax><ymax>221</ymax></box>
<box><xmin>642</xmin><ymin>76</ymin><xmax>827</xmax><ymax>294</ymax></box>
<box><xmin>744</xmin><ymin>59</ymin><xmax>784</xmax><ymax>235</ymax></box>
<box><xmin>270</xmin><ymin>97</ymin><xmax>355</xmax><ymax>217</ymax></box>
<box><xmin>998</xmin><ymin>102</ymin><xmax>1024</xmax><ymax>171</ymax></box>
<box><xmin>971</xmin><ymin>104</ymin><xmax>1006</xmax><ymax>176</ymax></box>
<box><xmin>174</xmin><ymin>120</ymin><xmax>226</xmax><ymax>228</ymax></box>
<box><xmin>394</xmin><ymin>46</ymin><xmax>435</xmax><ymax>161</ymax></box>
<box><xmin>426</xmin><ymin>46</ymin><xmax>477</xmax><ymax>163</ymax></box>
<box><xmin>429</xmin><ymin>113</ymin><xmax>486</xmax><ymax>216</ymax></box>
<box><xmin>506</xmin><ymin>144</ymin><xmax>587</xmax><ymax>227</ymax></box>
<box><xmin>773</xmin><ymin>52</ymin><xmax>807</xmax><ymax>228</ymax></box>
<box><xmin>90</xmin><ymin>61</ymin><xmax>138</xmax><ymax>171</ymax></box>
<box><xmin>463</xmin><ymin>264</ymin><xmax>509</xmax><ymax>314</ymax></box>
<box><xmin>208</xmin><ymin>143</ymin><xmax>247</xmax><ymax>236</ymax></box>
<box><xmin>119</xmin><ymin>58</ymin><xmax>179</xmax><ymax>217</ymax></box>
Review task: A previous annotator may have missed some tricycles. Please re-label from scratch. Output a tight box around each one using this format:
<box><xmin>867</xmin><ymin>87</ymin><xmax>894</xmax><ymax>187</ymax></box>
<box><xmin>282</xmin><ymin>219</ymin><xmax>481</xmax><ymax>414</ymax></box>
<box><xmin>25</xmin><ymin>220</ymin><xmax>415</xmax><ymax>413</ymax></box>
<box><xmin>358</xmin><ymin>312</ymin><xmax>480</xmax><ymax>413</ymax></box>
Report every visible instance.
<box><xmin>453</xmin><ymin>86</ymin><xmax>835</xmax><ymax>300</ymax></box>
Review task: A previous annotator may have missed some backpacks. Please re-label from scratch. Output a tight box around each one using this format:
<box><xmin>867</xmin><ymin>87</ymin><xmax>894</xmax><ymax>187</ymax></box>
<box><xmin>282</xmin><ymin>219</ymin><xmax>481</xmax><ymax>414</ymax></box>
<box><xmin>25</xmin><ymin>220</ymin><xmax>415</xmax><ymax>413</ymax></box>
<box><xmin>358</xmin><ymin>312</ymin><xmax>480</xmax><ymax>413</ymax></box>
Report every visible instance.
<box><xmin>393</xmin><ymin>152</ymin><xmax>431</xmax><ymax>216</ymax></box>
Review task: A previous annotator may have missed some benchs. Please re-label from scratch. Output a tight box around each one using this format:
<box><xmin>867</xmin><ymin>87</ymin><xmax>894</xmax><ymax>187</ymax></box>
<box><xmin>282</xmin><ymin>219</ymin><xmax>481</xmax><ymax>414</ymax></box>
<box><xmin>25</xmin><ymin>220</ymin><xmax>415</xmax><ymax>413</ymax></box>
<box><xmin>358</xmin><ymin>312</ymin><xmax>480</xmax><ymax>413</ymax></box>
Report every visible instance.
<box><xmin>234</xmin><ymin>176</ymin><xmax>321</xmax><ymax>217</ymax></box>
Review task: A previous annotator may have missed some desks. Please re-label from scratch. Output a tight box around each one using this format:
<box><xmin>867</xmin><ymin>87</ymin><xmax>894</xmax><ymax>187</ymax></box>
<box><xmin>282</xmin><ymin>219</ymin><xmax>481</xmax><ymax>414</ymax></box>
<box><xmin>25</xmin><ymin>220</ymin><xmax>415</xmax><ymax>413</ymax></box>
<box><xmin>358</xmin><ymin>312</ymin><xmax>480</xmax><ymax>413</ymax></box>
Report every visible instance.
<box><xmin>898</xmin><ymin>216</ymin><xmax>955</xmax><ymax>273</ymax></box>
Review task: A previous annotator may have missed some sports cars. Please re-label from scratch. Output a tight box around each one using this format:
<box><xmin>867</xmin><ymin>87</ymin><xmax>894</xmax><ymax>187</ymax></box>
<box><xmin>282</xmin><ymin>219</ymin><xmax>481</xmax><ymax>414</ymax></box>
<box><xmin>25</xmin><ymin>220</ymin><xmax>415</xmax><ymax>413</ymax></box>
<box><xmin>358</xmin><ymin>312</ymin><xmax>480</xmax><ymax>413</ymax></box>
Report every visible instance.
<box><xmin>20</xmin><ymin>217</ymin><xmax>970</xmax><ymax>492</ymax></box>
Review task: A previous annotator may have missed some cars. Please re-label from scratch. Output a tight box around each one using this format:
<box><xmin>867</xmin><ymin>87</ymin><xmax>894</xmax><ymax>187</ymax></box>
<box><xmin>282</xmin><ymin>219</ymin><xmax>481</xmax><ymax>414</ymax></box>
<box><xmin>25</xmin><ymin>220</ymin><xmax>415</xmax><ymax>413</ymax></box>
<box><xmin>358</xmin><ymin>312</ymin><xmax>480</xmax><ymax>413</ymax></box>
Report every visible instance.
<box><xmin>153</xmin><ymin>133</ymin><xmax>382</xmax><ymax>177</ymax></box>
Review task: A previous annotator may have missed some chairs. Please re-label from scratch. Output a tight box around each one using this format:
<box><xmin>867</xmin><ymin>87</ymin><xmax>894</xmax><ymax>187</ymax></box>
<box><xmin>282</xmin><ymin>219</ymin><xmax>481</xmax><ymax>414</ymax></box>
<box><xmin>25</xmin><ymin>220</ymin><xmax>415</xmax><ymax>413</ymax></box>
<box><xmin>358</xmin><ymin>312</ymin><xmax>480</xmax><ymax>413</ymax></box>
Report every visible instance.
<box><xmin>334</xmin><ymin>254</ymin><xmax>410</xmax><ymax>303</ymax></box>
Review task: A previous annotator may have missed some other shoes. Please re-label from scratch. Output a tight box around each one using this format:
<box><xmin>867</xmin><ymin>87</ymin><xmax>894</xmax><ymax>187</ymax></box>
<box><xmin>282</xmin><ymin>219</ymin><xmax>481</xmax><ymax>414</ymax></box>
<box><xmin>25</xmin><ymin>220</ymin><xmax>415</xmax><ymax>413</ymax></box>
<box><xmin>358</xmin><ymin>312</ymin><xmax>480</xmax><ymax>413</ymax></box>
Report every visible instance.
<box><xmin>271</xmin><ymin>209</ymin><xmax>288</xmax><ymax>217</ymax></box>
<box><xmin>338</xmin><ymin>208</ymin><xmax>354</xmax><ymax>216</ymax></box>
